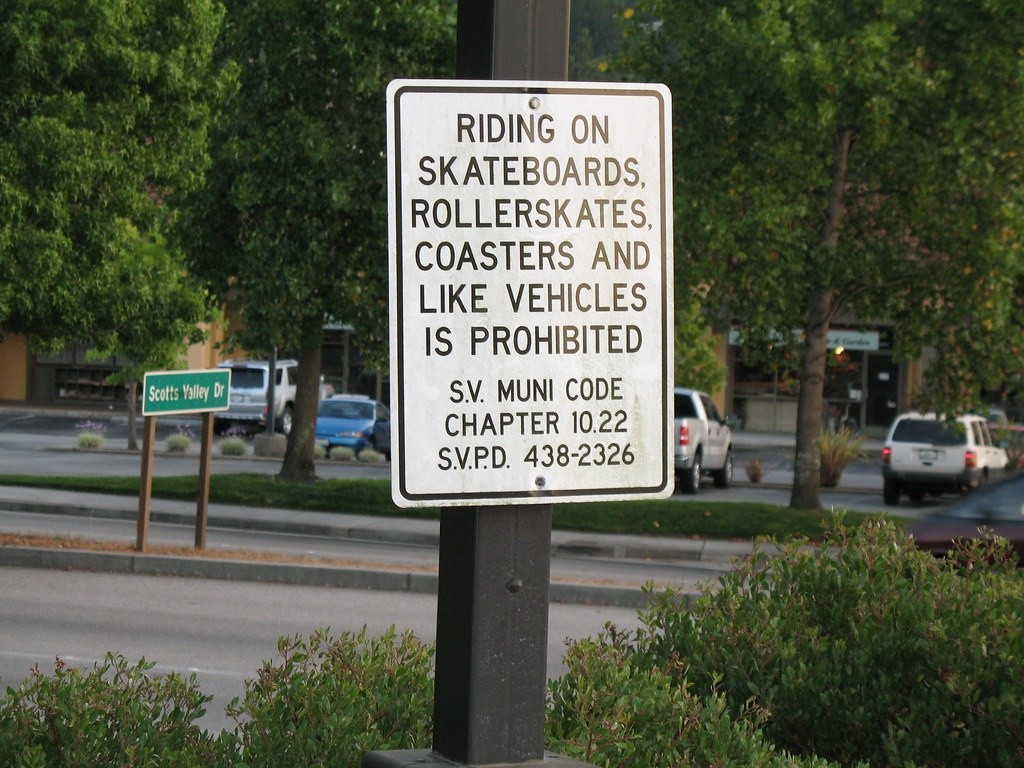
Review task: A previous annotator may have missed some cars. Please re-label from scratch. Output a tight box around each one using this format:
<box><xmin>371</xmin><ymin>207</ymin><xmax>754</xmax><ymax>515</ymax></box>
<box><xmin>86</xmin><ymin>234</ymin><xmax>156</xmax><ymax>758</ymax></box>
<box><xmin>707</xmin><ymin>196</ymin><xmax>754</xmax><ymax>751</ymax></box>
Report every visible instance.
<box><xmin>314</xmin><ymin>397</ymin><xmax>391</xmax><ymax>463</ymax></box>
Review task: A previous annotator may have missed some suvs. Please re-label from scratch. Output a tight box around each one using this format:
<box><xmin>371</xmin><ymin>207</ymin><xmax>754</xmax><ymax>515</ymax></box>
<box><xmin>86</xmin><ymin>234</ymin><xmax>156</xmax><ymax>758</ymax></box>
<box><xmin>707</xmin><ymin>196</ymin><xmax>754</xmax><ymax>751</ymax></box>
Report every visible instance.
<box><xmin>209</xmin><ymin>357</ymin><xmax>336</xmax><ymax>438</ymax></box>
<box><xmin>880</xmin><ymin>411</ymin><xmax>1010</xmax><ymax>506</ymax></box>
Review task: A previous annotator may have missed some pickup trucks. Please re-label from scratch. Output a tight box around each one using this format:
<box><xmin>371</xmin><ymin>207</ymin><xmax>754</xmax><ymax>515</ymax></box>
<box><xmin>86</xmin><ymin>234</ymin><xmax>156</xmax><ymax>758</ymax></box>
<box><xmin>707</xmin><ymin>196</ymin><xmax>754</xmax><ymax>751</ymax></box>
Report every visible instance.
<box><xmin>673</xmin><ymin>387</ymin><xmax>739</xmax><ymax>495</ymax></box>
